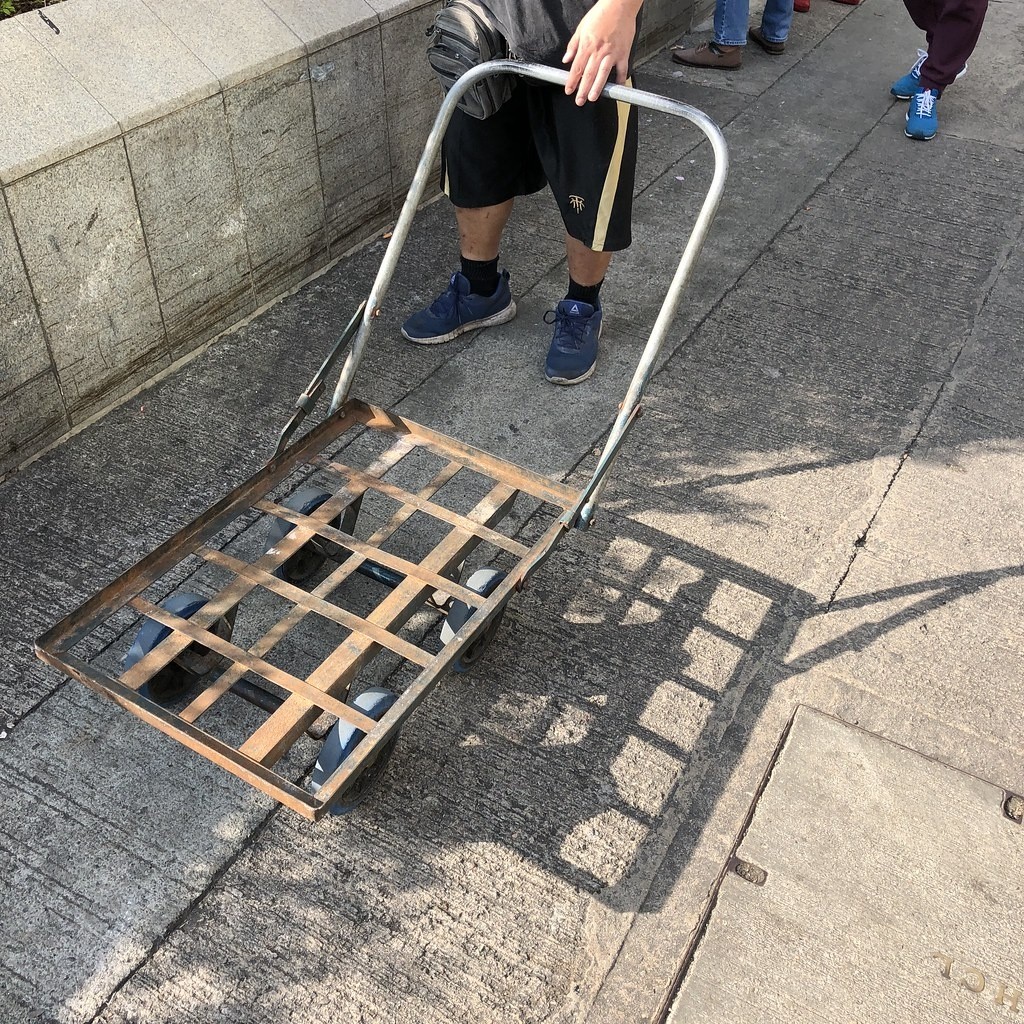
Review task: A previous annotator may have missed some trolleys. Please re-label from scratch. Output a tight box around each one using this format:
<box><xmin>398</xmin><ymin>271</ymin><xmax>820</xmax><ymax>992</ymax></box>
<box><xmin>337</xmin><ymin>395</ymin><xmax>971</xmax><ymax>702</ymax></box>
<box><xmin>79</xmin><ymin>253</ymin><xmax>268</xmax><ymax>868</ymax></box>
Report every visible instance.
<box><xmin>31</xmin><ymin>61</ymin><xmax>735</xmax><ymax>825</ymax></box>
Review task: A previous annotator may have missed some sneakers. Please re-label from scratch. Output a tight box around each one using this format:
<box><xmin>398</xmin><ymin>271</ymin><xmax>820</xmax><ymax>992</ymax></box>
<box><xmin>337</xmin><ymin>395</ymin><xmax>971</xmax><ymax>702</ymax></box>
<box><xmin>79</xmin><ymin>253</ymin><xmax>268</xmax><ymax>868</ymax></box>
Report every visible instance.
<box><xmin>673</xmin><ymin>43</ymin><xmax>743</xmax><ymax>70</ymax></box>
<box><xmin>904</xmin><ymin>87</ymin><xmax>939</xmax><ymax>140</ymax></box>
<box><xmin>750</xmin><ymin>25</ymin><xmax>785</xmax><ymax>54</ymax></box>
<box><xmin>543</xmin><ymin>294</ymin><xmax>602</xmax><ymax>389</ymax></box>
<box><xmin>401</xmin><ymin>269</ymin><xmax>516</xmax><ymax>345</ymax></box>
<box><xmin>891</xmin><ymin>49</ymin><xmax>967</xmax><ymax>99</ymax></box>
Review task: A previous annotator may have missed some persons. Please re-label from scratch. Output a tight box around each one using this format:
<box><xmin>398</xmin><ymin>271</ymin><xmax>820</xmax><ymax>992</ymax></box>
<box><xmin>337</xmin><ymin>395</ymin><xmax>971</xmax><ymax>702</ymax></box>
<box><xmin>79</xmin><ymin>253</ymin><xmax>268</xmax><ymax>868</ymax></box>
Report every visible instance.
<box><xmin>671</xmin><ymin>0</ymin><xmax>794</xmax><ymax>71</ymax></box>
<box><xmin>890</xmin><ymin>0</ymin><xmax>988</xmax><ymax>140</ymax></box>
<box><xmin>399</xmin><ymin>0</ymin><xmax>646</xmax><ymax>384</ymax></box>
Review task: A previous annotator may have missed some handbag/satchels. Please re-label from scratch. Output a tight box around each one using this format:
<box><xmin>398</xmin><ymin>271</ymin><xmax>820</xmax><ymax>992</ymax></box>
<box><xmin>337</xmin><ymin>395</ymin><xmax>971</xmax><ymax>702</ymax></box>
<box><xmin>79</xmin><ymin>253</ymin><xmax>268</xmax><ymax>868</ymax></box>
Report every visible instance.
<box><xmin>425</xmin><ymin>0</ymin><xmax>511</xmax><ymax>120</ymax></box>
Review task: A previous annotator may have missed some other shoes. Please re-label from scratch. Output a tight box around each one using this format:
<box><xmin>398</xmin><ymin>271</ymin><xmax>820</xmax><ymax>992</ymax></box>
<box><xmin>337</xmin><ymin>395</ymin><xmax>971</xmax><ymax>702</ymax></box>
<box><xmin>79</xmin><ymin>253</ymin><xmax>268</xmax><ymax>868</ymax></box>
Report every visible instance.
<box><xmin>793</xmin><ymin>1</ymin><xmax>809</xmax><ymax>13</ymax></box>
